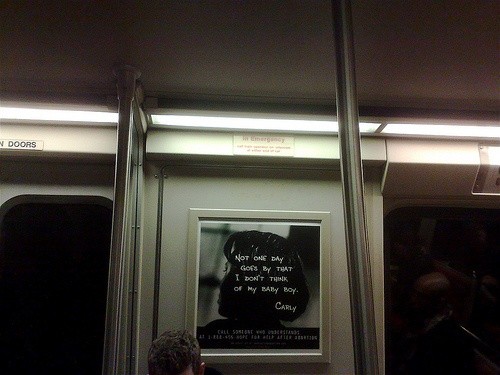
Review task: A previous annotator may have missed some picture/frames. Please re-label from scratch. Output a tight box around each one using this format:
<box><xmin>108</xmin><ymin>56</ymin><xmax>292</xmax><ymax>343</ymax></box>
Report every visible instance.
<box><xmin>183</xmin><ymin>207</ymin><xmax>334</xmax><ymax>366</ymax></box>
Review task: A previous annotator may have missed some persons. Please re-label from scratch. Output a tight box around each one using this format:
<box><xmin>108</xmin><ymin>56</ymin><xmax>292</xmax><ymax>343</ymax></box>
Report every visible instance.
<box><xmin>385</xmin><ymin>272</ymin><xmax>477</xmax><ymax>375</ymax></box>
<box><xmin>148</xmin><ymin>330</ymin><xmax>205</xmax><ymax>375</ymax></box>
<box><xmin>197</xmin><ymin>229</ymin><xmax>309</xmax><ymax>327</ymax></box>
